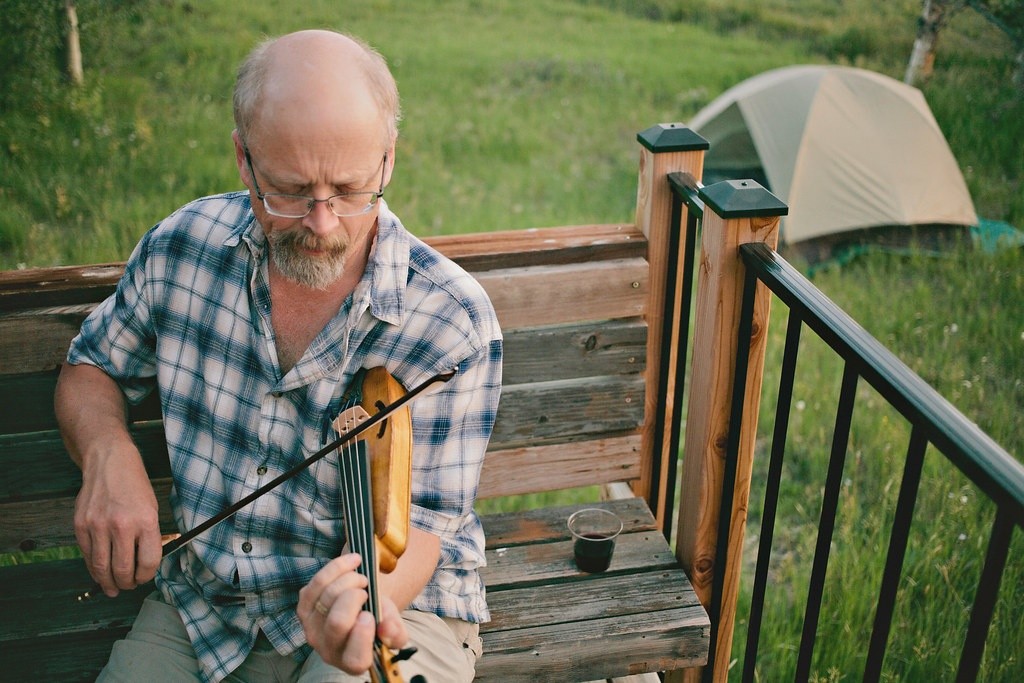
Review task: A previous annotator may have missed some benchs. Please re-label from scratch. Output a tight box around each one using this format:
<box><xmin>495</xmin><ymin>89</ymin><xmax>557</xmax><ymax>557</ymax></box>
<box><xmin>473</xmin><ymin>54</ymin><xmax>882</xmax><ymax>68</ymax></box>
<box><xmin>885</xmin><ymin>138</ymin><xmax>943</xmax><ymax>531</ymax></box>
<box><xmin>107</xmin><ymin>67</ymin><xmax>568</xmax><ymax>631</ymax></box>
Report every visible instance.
<box><xmin>0</xmin><ymin>256</ymin><xmax>711</xmax><ymax>683</ymax></box>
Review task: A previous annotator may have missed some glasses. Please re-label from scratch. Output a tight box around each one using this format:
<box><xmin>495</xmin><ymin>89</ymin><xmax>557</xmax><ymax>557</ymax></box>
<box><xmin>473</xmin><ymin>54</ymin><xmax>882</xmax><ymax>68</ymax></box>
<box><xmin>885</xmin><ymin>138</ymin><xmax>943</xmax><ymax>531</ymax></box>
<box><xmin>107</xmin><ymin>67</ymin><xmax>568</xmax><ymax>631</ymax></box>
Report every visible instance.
<box><xmin>244</xmin><ymin>141</ymin><xmax>387</xmax><ymax>218</ymax></box>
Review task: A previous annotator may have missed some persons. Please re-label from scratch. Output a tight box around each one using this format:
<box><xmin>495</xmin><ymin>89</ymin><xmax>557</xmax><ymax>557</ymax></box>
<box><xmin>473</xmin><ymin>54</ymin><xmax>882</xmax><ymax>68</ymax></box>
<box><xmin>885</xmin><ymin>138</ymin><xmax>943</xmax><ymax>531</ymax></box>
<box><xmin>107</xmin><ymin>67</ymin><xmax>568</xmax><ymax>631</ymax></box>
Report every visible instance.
<box><xmin>53</xmin><ymin>28</ymin><xmax>503</xmax><ymax>682</ymax></box>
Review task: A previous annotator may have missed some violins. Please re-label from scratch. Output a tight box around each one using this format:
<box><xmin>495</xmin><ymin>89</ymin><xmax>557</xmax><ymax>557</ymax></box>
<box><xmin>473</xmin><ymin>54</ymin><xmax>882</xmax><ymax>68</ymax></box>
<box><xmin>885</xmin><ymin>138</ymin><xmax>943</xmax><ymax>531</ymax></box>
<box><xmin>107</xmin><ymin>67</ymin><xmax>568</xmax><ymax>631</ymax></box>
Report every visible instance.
<box><xmin>329</xmin><ymin>364</ymin><xmax>415</xmax><ymax>683</ymax></box>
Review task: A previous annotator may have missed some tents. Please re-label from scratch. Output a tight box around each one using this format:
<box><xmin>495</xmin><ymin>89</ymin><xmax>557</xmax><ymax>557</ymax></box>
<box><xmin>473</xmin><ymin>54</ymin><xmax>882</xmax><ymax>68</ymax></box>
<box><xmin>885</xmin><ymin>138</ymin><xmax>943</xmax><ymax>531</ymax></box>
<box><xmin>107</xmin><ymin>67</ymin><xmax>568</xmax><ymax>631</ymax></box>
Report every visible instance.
<box><xmin>687</xmin><ymin>64</ymin><xmax>982</xmax><ymax>277</ymax></box>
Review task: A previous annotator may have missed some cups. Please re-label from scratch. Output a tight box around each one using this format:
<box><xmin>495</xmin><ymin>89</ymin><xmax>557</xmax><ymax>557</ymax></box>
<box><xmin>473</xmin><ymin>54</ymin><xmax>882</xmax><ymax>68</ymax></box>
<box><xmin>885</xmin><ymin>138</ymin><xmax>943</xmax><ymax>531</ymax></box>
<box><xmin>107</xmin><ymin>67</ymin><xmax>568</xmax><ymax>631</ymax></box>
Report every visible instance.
<box><xmin>568</xmin><ymin>508</ymin><xmax>625</xmax><ymax>574</ymax></box>
